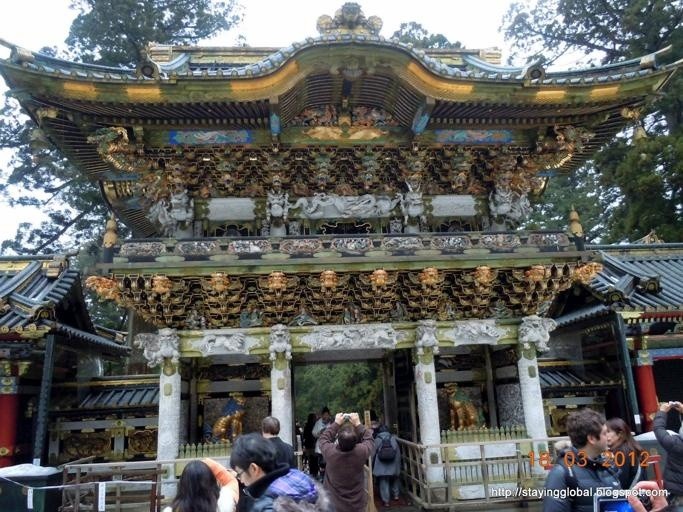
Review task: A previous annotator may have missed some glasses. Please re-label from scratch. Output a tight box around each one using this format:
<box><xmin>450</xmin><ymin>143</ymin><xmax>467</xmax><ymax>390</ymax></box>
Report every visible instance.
<box><xmin>235</xmin><ymin>470</ymin><xmax>244</xmax><ymax>481</ymax></box>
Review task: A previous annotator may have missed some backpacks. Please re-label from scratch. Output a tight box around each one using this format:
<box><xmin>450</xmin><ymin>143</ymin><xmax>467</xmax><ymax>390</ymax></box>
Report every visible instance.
<box><xmin>375</xmin><ymin>434</ymin><xmax>395</xmax><ymax>464</ymax></box>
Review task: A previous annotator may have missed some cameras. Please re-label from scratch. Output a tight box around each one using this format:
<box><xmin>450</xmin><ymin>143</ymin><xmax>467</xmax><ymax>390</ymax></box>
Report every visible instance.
<box><xmin>669</xmin><ymin>401</ymin><xmax>679</xmax><ymax>408</ymax></box>
<box><xmin>595</xmin><ymin>492</ymin><xmax>651</xmax><ymax>511</ymax></box>
<box><xmin>343</xmin><ymin>414</ymin><xmax>351</xmax><ymax>424</ymax></box>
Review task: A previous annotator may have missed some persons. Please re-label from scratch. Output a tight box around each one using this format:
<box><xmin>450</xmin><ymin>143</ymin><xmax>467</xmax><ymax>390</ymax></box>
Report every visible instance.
<box><xmin>363</xmin><ymin>409</ymin><xmax>383</xmax><ymax>496</ymax></box>
<box><xmin>626</xmin><ymin>481</ymin><xmax>683</xmax><ymax>511</ymax></box>
<box><xmin>311</xmin><ymin>406</ymin><xmax>335</xmax><ymax>484</ymax></box>
<box><xmin>229</xmin><ymin>432</ymin><xmax>335</xmax><ymax>512</ymax></box>
<box><xmin>542</xmin><ymin>406</ymin><xmax>626</xmax><ymax>511</ymax></box>
<box><xmin>162</xmin><ymin>456</ymin><xmax>240</xmax><ymax>511</ymax></box>
<box><xmin>604</xmin><ymin>418</ymin><xmax>650</xmax><ymax>490</ymax></box>
<box><xmin>372</xmin><ymin>424</ymin><xmax>402</xmax><ymax>508</ymax></box>
<box><xmin>261</xmin><ymin>417</ymin><xmax>296</xmax><ymax>469</ymax></box>
<box><xmin>652</xmin><ymin>401</ymin><xmax>682</xmax><ymax>494</ymax></box>
<box><xmin>319</xmin><ymin>412</ymin><xmax>377</xmax><ymax>511</ymax></box>
<box><xmin>302</xmin><ymin>413</ymin><xmax>319</xmax><ymax>477</ymax></box>
<box><xmin>295</xmin><ymin>419</ymin><xmax>308</xmax><ymax>463</ymax></box>
